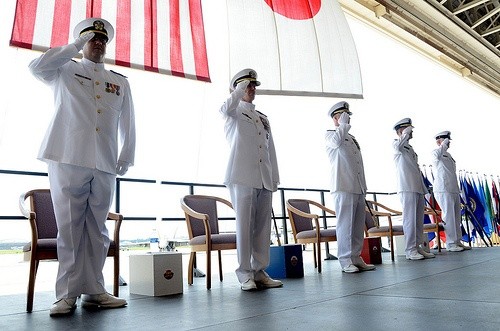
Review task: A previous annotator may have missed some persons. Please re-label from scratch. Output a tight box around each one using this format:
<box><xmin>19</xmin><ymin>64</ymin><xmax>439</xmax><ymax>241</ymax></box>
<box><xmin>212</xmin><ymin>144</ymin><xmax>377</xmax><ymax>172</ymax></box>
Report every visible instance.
<box><xmin>392</xmin><ymin>118</ymin><xmax>435</xmax><ymax>260</ymax></box>
<box><xmin>221</xmin><ymin>69</ymin><xmax>283</xmax><ymax>291</ymax></box>
<box><xmin>28</xmin><ymin>18</ymin><xmax>135</xmax><ymax>314</ymax></box>
<box><xmin>431</xmin><ymin>130</ymin><xmax>471</xmax><ymax>252</ymax></box>
<box><xmin>323</xmin><ymin>102</ymin><xmax>376</xmax><ymax>273</ymax></box>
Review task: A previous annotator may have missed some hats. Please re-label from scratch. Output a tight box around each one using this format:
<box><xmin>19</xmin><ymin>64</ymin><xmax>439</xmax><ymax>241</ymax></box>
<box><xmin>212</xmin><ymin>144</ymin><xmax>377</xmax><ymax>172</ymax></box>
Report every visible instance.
<box><xmin>328</xmin><ymin>101</ymin><xmax>352</xmax><ymax>119</ymax></box>
<box><xmin>230</xmin><ymin>68</ymin><xmax>260</xmax><ymax>90</ymax></box>
<box><xmin>73</xmin><ymin>17</ymin><xmax>114</xmax><ymax>45</ymax></box>
<box><xmin>434</xmin><ymin>131</ymin><xmax>452</xmax><ymax>141</ymax></box>
<box><xmin>393</xmin><ymin>118</ymin><xmax>415</xmax><ymax>131</ymax></box>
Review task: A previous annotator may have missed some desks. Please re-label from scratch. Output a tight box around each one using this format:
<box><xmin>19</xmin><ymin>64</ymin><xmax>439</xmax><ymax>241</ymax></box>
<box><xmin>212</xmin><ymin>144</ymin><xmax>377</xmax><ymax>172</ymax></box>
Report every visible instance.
<box><xmin>129</xmin><ymin>253</ymin><xmax>183</xmax><ymax>296</ymax></box>
<box><xmin>360</xmin><ymin>236</ymin><xmax>382</xmax><ymax>264</ymax></box>
<box><xmin>265</xmin><ymin>244</ymin><xmax>304</xmax><ymax>279</ymax></box>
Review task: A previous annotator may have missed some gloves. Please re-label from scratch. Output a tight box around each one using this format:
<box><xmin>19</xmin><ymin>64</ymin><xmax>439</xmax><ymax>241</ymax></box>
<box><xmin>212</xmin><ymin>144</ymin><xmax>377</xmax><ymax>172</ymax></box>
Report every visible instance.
<box><xmin>402</xmin><ymin>126</ymin><xmax>414</xmax><ymax>135</ymax></box>
<box><xmin>73</xmin><ymin>31</ymin><xmax>95</xmax><ymax>51</ymax></box>
<box><xmin>115</xmin><ymin>160</ymin><xmax>130</xmax><ymax>176</ymax></box>
<box><xmin>236</xmin><ymin>80</ymin><xmax>250</xmax><ymax>94</ymax></box>
<box><xmin>338</xmin><ymin>112</ymin><xmax>350</xmax><ymax>125</ymax></box>
<box><xmin>444</xmin><ymin>138</ymin><xmax>449</xmax><ymax>145</ymax></box>
<box><xmin>272</xmin><ymin>183</ymin><xmax>278</xmax><ymax>192</ymax></box>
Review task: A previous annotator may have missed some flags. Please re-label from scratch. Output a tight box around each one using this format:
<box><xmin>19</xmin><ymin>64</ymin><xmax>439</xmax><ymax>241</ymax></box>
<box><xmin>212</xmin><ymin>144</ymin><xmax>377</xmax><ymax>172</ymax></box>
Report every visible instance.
<box><xmin>8</xmin><ymin>0</ymin><xmax>211</xmax><ymax>83</ymax></box>
<box><xmin>226</xmin><ymin>0</ymin><xmax>364</xmax><ymax>99</ymax></box>
<box><xmin>419</xmin><ymin>165</ymin><xmax>500</xmax><ymax>246</ymax></box>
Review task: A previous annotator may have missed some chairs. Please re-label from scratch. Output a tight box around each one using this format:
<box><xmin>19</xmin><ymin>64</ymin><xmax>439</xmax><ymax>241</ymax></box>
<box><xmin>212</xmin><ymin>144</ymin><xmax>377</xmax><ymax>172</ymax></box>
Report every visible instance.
<box><xmin>287</xmin><ymin>198</ymin><xmax>335</xmax><ymax>273</ymax></box>
<box><xmin>363</xmin><ymin>200</ymin><xmax>404</xmax><ymax>260</ymax></box>
<box><xmin>180</xmin><ymin>194</ymin><xmax>236</xmax><ymax>290</ymax></box>
<box><xmin>422</xmin><ymin>205</ymin><xmax>444</xmax><ymax>252</ymax></box>
<box><xmin>19</xmin><ymin>189</ymin><xmax>123</xmax><ymax>313</ymax></box>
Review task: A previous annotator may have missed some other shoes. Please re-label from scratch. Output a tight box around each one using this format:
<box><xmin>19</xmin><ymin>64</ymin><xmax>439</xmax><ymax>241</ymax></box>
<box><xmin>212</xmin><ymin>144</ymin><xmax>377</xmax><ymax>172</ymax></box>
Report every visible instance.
<box><xmin>458</xmin><ymin>242</ymin><xmax>472</xmax><ymax>250</ymax></box>
<box><xmin>353</xmin><ymin>262</ymin><xmax>376</xmax><ymax>271</ymax></box>
<box><xmin>341</xmin><ymin>264</ymin><xmax>359</xmax><ymax>273</ymax></box>
<box><xmin>254</xmin><ymin>276</ymin><xmax>283</xmax><ymax>288</ymax></box>
<box><xmin>405</xmin><ymin>252</ymin><xmax>425</xmax><ymax>260</ymax></box>
<box><xmin>49</xmin><ymin>296</ymin><xmax>78</xmax><ymax>317</ymax></box>
<box><xmin>419</xmin><ymin>249</ymin><xmax>435</xmax><ymax>259</ymax></box>
<box><xmin>81</xmin><ymin>292</ymin><xmax>127</xmax><ymax>311</ymax></box>
<box><xmin>445</xmin><ymin>244</ymin><xmax>464</xmax><ymax>252</ymax></box>
<box><xmin>241</xmin><ymin>279</ymin><xmax>257</xmax><ymax>290</ymax></box>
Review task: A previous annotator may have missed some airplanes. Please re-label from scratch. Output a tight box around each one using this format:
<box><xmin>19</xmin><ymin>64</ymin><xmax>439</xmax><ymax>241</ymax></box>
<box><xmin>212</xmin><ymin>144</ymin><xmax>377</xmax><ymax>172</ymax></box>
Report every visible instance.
<box><xmin>121</xmin><ymin>237</ymin><xmax>191</xmax><ymax>252</ymax></box>
